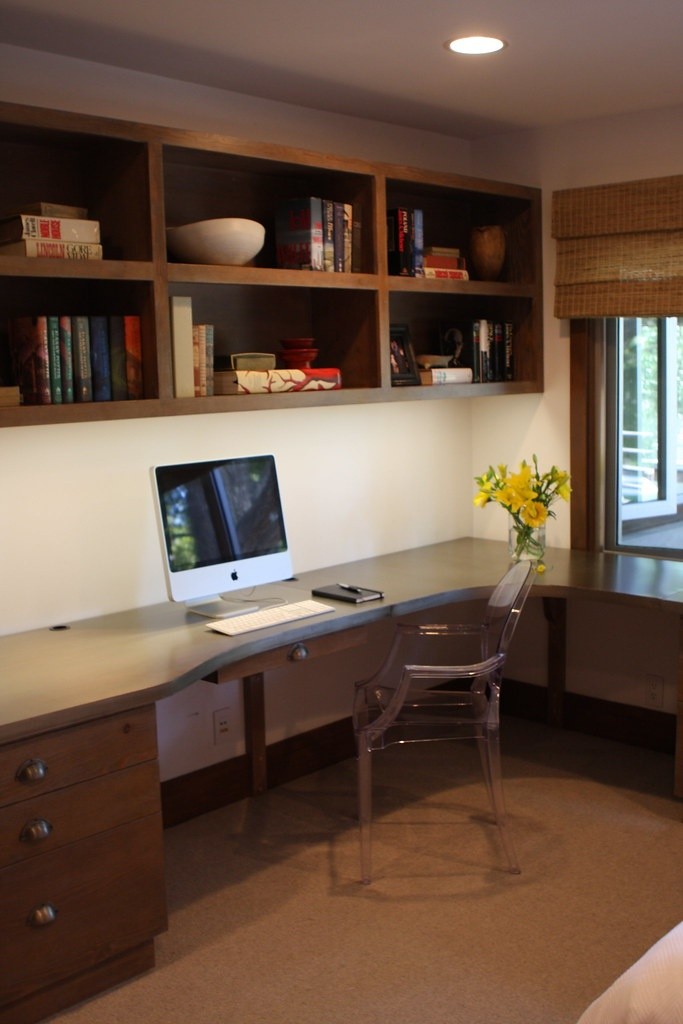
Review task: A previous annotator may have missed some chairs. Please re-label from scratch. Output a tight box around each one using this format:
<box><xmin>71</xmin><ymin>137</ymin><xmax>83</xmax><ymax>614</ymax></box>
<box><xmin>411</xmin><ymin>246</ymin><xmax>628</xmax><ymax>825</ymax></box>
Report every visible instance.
<box><xmin>344</xmin><ymin>555</ymin><xmax>547</xmax><ymax>887</ymax></box>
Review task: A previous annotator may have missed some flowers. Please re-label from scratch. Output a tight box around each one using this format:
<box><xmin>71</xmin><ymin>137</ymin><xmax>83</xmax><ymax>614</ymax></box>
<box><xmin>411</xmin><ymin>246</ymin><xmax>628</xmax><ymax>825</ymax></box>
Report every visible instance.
<box><xmin>473</xmin><ymin>452</ymin><xmax>572</xmax><ymax>575</ymax></box>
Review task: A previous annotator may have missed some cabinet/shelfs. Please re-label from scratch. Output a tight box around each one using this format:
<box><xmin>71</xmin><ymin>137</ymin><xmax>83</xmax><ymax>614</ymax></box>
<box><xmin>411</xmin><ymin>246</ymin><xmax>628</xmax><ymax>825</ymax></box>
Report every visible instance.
<box><xmin>0</xmin><ymin>698</ymin><xmax>172</xmax><ymax>1024</ymax></box>
<box><xmin>0</xmin><ymin>97</ymin><xmax>544</xmax><ymax>427</ymax></box>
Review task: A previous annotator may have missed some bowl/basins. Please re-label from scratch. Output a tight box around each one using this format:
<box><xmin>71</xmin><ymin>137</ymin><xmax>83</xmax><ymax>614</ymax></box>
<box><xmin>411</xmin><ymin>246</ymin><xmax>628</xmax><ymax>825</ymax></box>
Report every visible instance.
<box><xmin>166</xmin><ymin>217</ymin><xmax>266</xmax><ymax>267</ymax></box>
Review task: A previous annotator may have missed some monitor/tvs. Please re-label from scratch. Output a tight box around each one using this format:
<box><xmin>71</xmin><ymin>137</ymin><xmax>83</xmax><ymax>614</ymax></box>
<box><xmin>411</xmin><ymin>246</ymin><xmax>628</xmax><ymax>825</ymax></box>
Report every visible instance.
<box><xmin>148</xmin><ymin>454</ymin><xmax>295</xmax><ymax>603</ymax></box>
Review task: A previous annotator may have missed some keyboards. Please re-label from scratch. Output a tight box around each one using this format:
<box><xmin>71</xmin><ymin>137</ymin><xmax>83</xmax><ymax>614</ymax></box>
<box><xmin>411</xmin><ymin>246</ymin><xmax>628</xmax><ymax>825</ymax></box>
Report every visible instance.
<box><xmin>204</xmin><ymin>600</ymin><xmax>335</xmax><ymax>637</ymax></box>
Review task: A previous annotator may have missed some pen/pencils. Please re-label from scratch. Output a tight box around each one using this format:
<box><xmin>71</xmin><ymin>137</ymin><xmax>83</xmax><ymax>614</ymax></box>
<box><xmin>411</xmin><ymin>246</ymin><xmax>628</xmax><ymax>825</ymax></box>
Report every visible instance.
<box><xmin>337</xmin><ymin>582</ymin><xmax>363</xmax><ymax>594</ymax></box>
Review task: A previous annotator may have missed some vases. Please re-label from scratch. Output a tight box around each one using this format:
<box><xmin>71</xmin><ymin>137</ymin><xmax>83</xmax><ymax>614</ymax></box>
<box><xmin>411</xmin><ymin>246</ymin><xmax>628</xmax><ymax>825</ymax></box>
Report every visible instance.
<box><xmin>507</xmin><ymin>509</ymin><xmax>551</xmax><ymax>562</ymax></box>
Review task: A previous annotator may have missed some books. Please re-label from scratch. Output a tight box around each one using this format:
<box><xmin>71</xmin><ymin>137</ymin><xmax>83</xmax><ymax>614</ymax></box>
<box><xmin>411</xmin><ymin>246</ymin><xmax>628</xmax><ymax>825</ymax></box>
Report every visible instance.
<box><xmin>414</xmin><ymin>209</ymin><xmax>425</xmax><ymax>277</ymax></box>
<box><xmin>1</xmin><ymin>241</ymin><xmax>103</xmax><ymax>259</ymax></box>
<box><xmin>214</xmin><ymin>368</ymin><xmax>348</xmax><ymax>392</ymax></box>
<box><xmin>353</xmin><ymin>203</ymin><xmax>362</xmax><ymax>273</ymax></box>
<box><xmin>479</xmin><ymin>318</ymin><xmax>489</xmax><ymax>383</ymax></box>
<box><xmin>343</xmin><ymin>204</ymin><xmax>353</xmax><ymax>273</ymax></box>
<box><xmin>206</xmin><ymin>324</ymin><xmax>218</xmax><ymax>396</ymax></box>
<box><xmin>125</xmin><ymin>315</ymin><xmax>147</xmax><ymax>401</ymax></box>
<box><xmin>487</xmin><ymin>322</ymin><xmax>496</xmax><ymax>383</ymax></box>
<box><xmin>322</xmin><ymin>199</ymin><xmax>336</xmax><ymax>273</ymax></box>
<box><xmin>59</xmin><ymin>316</ymin><xmax>78</xmax><ymax>404</ymax></box>
<box><xmin>503</xmin><ymin>324</ymin><xmax>514</xmax><ymax>380</ymax></box>
<box><xmin>420</xmin><ymin>364</ymin><xmax>474</xmax><ymax>385</ymax></box>
<box><xmin>360</xmin><ymin>182</ymin><xmax>374</xmax><ymax>275</ymax></box>
<box><xmin>48</xmin><ymin>315</ymin><xmax>62</xmax><ymax>404</ymax></box>
<box><xmin>71</xmin><ymin>316</ymin><xmax>96</xmax><ymax>403</ymax></box>
<box><xmin>0</xmin><ymin>383</ymin><xmax>22</xmax><ymax>406</ymax></box>
<box><xmin>13</xmin><ymin>200</ymin><xmax>88</xmax><ymax>220</ymax></box>
<box><xmin>168</xmin><ymin>295</ymin><xmax>196</xmax><ymax>398</ymax></box>
<box><xmin>495</xmin><ymin>324</ymin><xmax>505</xmax><ymax>382</ymax></box>
<box><xmin>199</xmin><ymin>324</ymin><xmax>209</xmax><ymax>396</ymax></box>
<box><xmin>426</xmin><ymin>241</ymin><xmax>469</xmax><ymax>257</ymax></box>
<box><xmin>433</xmin><ymin>320</ymin><xmax>479</xmax><ymax>384</ymax></box>
<box><xmin>3</xmin><ymin>311</ymin><xmax>51</xmax><ymax>404</ymax></box>
<box><xmin>272</xmin><ymin>195</ymin><xmax>324</xmax><ymax>271</ymax></box>
<box><xmin>106</xmin><ymin>316</ymin><xmax>127</xmax><ymax>400</ymax></box>
<box><xmin>88</xmin><ymin>315</ymin><xmax>115</xmax><ymax>402</ymax></box>
<box><xmin>332</xmin><ymin>202</ymin><xmax>343</xmax><ymax>273</ymax></box>
<box><xmin>385</xmin><ymin>181</ymin><xmax>415</xmax><ymax>278</ymax></box>
<box><xmin>421</xmin><ymin>266</ymin><xmax>470</xmax><ymax>282</ymax></box>
<box><xmin>422</xmin><ymin>253</ymin><xmax>469</xmax><ymax>271</ymax></box>
<box><xmin>192</xmin><ymin>324</ymin><xmax>205</xmax><ymax>397</ymax></box>
<box><xmin>311</xmin><ymin>580</ymin><xmax>387</xmax><ymax>604</ymax></box>
<box><xmin>0</xmin><ymin>212</ymin><xmax>101</xmax><ymax>247</ymax></box>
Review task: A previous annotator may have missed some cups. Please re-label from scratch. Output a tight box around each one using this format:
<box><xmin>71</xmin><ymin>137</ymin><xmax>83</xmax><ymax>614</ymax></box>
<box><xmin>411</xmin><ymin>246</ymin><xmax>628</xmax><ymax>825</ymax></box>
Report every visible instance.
<box><xmin>280</xmin><ymin>336</ymin><xmax>313</xmax><ymax>349</ymax></box>
<box><xmin>469</xmin><ymin>224</ymin><xmax>505</xmax><ymax>282</ymax></box>
<box><xmin>280</xmin><ymin>347</ymin><xmax>320</xmax><ymax>370</ymax></box>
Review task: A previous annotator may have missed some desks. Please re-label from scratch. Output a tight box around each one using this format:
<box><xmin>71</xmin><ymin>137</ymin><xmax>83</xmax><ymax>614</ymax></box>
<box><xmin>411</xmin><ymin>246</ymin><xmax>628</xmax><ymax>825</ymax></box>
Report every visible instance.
<box><xmin>0</xmin><ymin>535</ymin><xmax>683</xmax><ymax>740</ymax></box>
